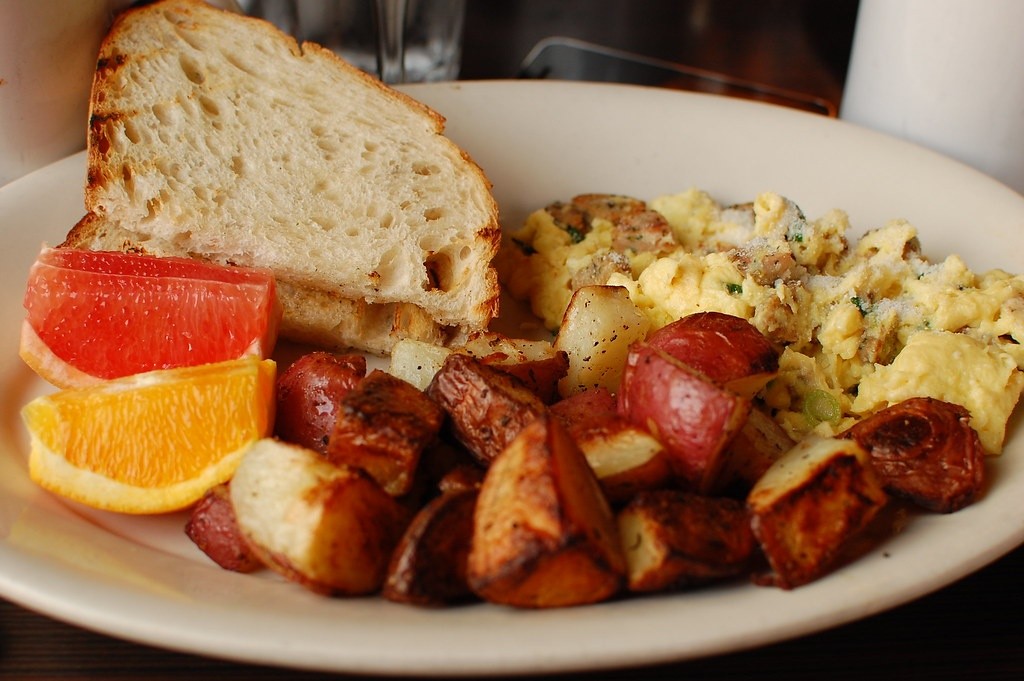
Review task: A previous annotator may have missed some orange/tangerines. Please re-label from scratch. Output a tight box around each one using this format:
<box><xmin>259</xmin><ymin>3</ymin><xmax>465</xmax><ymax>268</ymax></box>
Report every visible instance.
<box><xmin>17</xmin><ymin>244</ymin><xmax>285</xmax><ymax>391</ymax></box>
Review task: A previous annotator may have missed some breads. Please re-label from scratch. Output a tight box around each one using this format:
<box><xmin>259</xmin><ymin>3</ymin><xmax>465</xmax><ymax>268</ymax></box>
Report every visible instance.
<box><xmin>54</xmin><ymin>1</ymin><xmax>506</xmax><ymax>356</ymax></box>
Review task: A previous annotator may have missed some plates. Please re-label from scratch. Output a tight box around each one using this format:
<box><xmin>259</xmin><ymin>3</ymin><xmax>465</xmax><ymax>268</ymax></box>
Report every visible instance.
<box><xmin>0</xmin><ymin>78</ymin><xmax>1024</xmax><ymax>676</ymax></box>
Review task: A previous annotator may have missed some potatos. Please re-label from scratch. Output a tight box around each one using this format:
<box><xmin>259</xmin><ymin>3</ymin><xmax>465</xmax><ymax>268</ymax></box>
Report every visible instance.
<box><xmin>185</xmin><ymin>285</ymin><xmax>985</xmax><ymax>611</ymax></box>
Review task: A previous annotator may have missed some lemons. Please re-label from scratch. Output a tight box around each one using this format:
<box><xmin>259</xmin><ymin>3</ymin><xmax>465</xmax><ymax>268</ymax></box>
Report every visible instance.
<box><xmin>21</xmin><ymin>356</ymin><xmax>274</xmax><ymax>514</ymax></box>
